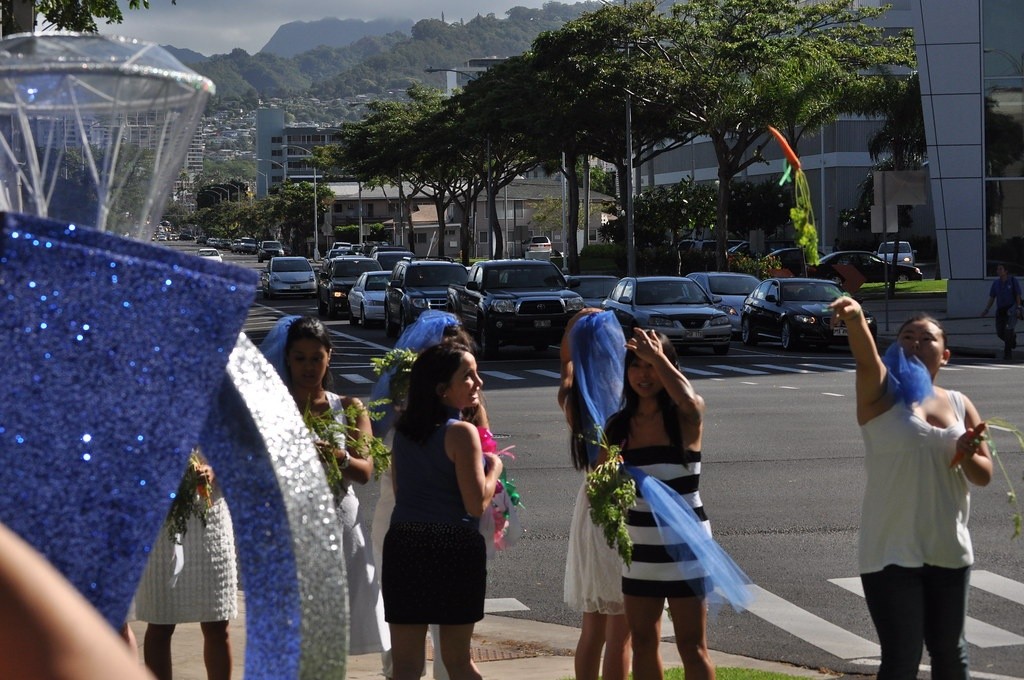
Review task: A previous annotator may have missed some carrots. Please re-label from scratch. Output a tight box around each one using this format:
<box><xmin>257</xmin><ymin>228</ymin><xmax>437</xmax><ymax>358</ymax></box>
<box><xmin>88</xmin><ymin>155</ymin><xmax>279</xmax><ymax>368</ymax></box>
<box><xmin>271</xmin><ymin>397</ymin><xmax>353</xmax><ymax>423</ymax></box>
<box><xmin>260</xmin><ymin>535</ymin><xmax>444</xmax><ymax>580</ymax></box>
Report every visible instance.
<box><xmin>950</xmin><ymin>422</ymin><xmax>985</xmax><ymax>466</ymax></box>
<box><xmin>198</xmin><ymin>483</ymin><xmax>211</xmax><ymax>508</ymax></box>
<box><xmin>767</xmin><ymin>124</ymin><xmax>801</xmax><ymax>169</ymax></box>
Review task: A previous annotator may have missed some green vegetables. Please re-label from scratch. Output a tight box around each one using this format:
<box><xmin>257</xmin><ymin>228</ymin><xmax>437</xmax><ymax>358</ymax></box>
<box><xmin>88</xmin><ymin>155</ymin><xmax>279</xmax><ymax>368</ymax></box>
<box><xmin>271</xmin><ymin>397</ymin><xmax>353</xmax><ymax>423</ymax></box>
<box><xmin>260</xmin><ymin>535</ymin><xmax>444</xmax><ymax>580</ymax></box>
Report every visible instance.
<box><xmin>577</xmin><ymin>424</ymin><xmax>636</xmax><ymax>571</ymax></box>
<box><xmin>370</xmin><ymin>346</ymin><xmax>417</xmax><ymax>406</ymax></box>
<box><xmin>301</xmin><ymin>394</ymin><xmax>391</xmax><ymax>485</ymax></box>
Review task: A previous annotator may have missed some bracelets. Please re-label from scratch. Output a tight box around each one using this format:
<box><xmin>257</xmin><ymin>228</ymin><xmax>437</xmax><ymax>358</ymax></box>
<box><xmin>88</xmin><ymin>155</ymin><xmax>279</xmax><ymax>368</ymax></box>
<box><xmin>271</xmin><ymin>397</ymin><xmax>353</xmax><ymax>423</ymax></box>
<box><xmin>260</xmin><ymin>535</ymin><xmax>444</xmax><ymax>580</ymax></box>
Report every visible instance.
<box><xmin>338</xmin><ymin>450</ymin><xmax>350</xmax><ymax>469</ymax></box>
<box><xmin>843</xmin><ymin>310</ymin><xmax>860</xmax><ymax>320</ymax></box>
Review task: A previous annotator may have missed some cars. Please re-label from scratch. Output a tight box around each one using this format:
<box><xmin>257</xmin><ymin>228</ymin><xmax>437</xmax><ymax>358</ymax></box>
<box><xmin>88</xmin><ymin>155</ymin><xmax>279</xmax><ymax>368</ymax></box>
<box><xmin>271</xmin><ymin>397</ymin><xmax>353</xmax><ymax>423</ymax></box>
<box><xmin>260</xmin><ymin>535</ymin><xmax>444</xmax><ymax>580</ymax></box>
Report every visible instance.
<box><xmin>347</xmin><ymin>270</ymin><xmax>394</xmax><ymax>329</ymax></box>
<box><xmin>739</xmin><ymin>277</ymin><xmax>879</xmax><ymax>352</ymax></box>
<box><xmin>681</xmin><ymin>270</ymin><xmax>761</xmax><ymax>332</ymax></box>
<box><xmin>261</xmin><ymin>255</ymin><xmax>317</xmax><ymax>300</ymax></box>
<box><xmin>599</xmin><ymin>274</ymin><xmax>732</xmax><ymax>355</ymax></box>
<box><xmin>195</xmin><ymin>248</ymin><xmax>224</xmax><ymax>263</ymax></box>
<box><xmin>676</xmin><ymin>238</ymin><xmax>826</xmax><ymax>277</ymax></box>
<box><xmin>874</xmin><ymin>240</ymin><xmax>918</xmax><ymax>267</ymax></box>
<box><xmin>315</xmin><ymin>241</ymin><xmax>416</xmax><ymax>316</ymax></box>
<box><xmin>564</xmin><ymin>275</ymin><xmax>620</xmax><ymax>309</ymax></box>
<box><xmin>526</xmin><ymin>236</ymin><xmax>553</xmax><ymax>253</ymax></box>
<box><xmin>804</xmin><ymin>250</ymin><xmax>923</xmax><ymax>284</ymax></box>
<box><xmin>154</xmin><ymin>229</ymin><xmax>258</xmax><ymax>255</ymax></box>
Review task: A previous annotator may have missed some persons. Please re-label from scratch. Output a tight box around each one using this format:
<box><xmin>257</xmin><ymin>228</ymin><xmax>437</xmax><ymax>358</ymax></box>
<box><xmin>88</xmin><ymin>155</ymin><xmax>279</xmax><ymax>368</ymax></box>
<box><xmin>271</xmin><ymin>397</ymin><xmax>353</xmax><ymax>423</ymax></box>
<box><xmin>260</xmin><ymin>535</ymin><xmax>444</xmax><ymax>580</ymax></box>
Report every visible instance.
<box><xmin>380</xmin><ymin>322</ymin><xmax>502</xmax><ymax>680</ymax></box>
<box><xmin>1</xmin><ymin>522</ymin><xmax>161</xmax><ymax>680</ymax></box>
<box><xmin>981</xmin><ymin>262</ymin><xmax>1024</xmax><ymax>363</ymax></box>
<box><xmin>280</xmin><ymin>313</ymin><xmax>393</xmax><ymax>658</ymax></box>
<box><xmin>132</xmin><ymin>442</ymin><xmax>239</xmax><ymax>680</ymax></box>
<box><xmin>828</xmin><ymin>297</ymin><xmax>993</xmax><ymax>680</ymax></box>
<box><xmin>558</xmin><ymin>309</ymin><xmax>716</xmax><ymax>680</ymax></box>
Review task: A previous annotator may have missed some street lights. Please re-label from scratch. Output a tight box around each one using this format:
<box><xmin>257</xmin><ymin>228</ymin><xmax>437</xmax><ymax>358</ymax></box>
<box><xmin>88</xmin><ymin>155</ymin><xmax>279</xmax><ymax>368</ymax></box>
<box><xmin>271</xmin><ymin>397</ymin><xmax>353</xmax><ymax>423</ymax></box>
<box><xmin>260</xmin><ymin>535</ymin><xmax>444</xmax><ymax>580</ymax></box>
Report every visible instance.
<box><xmin>204</xmin><ymin>189</ymin><xmax>223</xmax><ymax>202</ymax></box>
<box><xmin>219</xmin><ymin>182</ymin><xmax>242</xmax><ymax>232</ymax></box>
<box><xmin>211</xmin><ymin>186</ymin><xmax>230</xmax><ymax>202</ymax></box>
<box><xmin>242</xmin><ymin>168</ymin><xmax>269</xmax><ymax>197</ymax></box>
<box><xmin>279</xmin><ymin>144</ymin><xmax>318</xmax><ymax>263</ymax></box>
<box><xmin>256</xmin><ymin>158</ymin><xmax>286</xmax><ymax>182</ymax></box>
<box><xmin>422</xmin><ymin>65</ymin><xmax>494</xmax><ymax>260</ymax></box>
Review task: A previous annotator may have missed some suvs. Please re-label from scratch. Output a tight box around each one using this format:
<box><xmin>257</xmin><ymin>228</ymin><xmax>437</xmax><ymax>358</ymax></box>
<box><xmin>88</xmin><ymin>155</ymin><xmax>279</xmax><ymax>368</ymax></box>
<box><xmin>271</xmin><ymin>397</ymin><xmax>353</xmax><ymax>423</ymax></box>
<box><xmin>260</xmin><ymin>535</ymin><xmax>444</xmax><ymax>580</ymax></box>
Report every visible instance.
<box><xmin>256</xmin><ymin>240</ymin><xmax>284</xmax><ymax>263</ymax></box>
<box><xmin>385</xmin><ymin>255</ymin><xmax>468</xmax><ymax>337</ymax></box>
<box><xmin>446</xmin><ymin>258</ymin><xmax>585</xmax><ymax>359</ymax></box>
